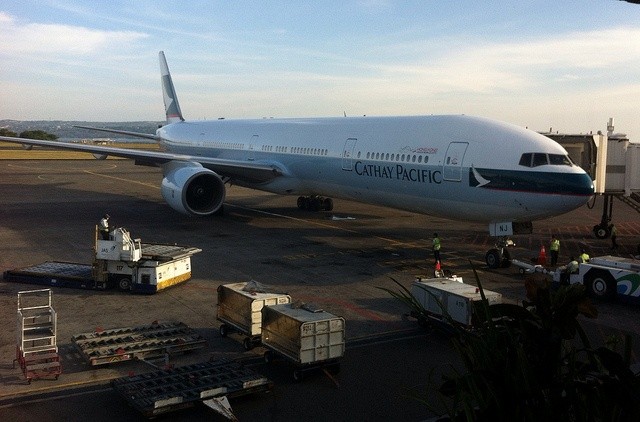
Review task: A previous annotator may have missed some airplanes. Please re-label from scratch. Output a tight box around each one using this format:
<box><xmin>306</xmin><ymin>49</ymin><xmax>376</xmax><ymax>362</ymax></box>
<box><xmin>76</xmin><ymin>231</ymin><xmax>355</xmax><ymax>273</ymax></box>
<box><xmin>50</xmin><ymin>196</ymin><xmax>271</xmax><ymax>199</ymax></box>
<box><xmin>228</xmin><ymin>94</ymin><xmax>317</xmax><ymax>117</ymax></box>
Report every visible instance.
<box><xmin>0</xmin><ymin>51</ymin><xmax>595</xmax><ymax>268</ymax></box>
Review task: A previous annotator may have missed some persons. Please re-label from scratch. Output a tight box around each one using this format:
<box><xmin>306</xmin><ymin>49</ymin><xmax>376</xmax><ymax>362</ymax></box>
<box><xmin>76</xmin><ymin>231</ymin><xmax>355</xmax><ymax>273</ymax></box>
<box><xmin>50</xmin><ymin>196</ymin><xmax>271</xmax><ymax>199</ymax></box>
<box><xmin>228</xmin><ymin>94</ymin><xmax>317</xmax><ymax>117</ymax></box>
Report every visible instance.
<box><xmin>546</xmin><ymin>235</ymin><xmax>561</xmax><ymax>267</ymax></box>
<box><xmin>98</xmin><ymin>213</ymin><xmax>116</xmax><ymax>241</ymax></box>
<box><xmin>578</xmin><ymin>248</ymin><xmax>590</xmax><ymax>263</ymax></box>
<box><xmin>565</xmin><ymin>255</ymin><xmax>579</xmax><ymax>284</ymax></box>
<box><xmin>431</xmin><ymin>232</ymin><xmax>442</xmax><ymax>265</ymax></box>
